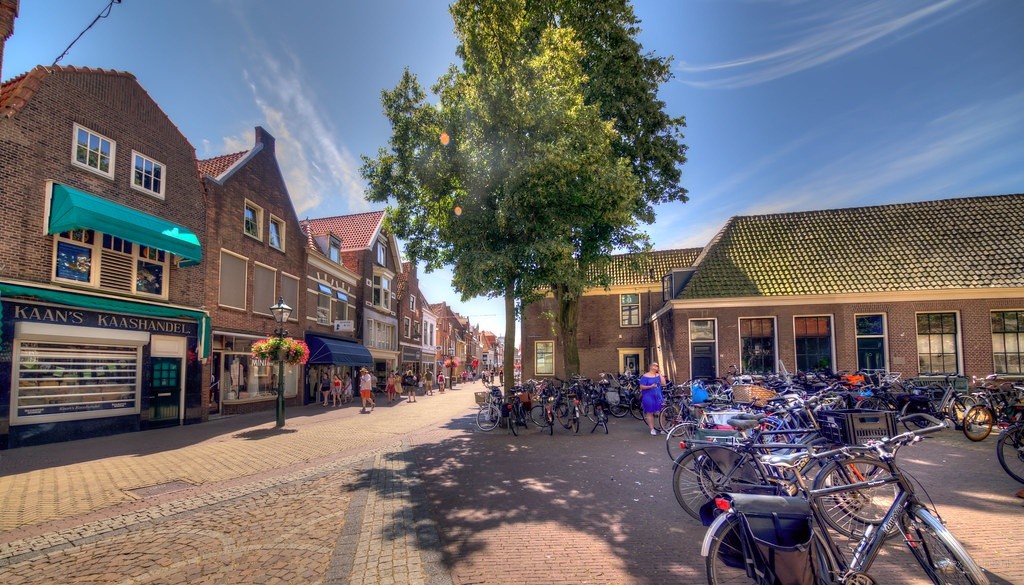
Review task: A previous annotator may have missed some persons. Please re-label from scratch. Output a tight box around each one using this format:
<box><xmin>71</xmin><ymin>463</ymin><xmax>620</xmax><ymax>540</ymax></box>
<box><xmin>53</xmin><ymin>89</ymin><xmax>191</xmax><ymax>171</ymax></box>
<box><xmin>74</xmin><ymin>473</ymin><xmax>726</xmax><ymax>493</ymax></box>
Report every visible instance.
<box><xmin>462</xmin><ymin>370</ymin><xmax>489</xmax><ymax>384</ymax></box>
<box><xmin>437</xmin><ymin>371</ymin><xmax>445</xmax><ymax>391</ymax></box>
<box><xmin>317</xmin><ymin>368</ymin><xmax>352</xmax><ymax>408</ymax></box>
<box><xmin>358</xmin><ymin>367</ymin><xmax>377</xmax><ymax>410</ymax></box>
<box><xmin>385</xmin><ymin>367</ymin><xmax>417</xmax><ymax>403</ymax></box>
<box><xmin>640</xmin><ymin>362</ymin><xmax>668</xmax><ymax>435</ymax></box>
<box><xmin>419</xmin><ymin>369</ymin><xmax>433</xmax><ymax>396</ymax></box>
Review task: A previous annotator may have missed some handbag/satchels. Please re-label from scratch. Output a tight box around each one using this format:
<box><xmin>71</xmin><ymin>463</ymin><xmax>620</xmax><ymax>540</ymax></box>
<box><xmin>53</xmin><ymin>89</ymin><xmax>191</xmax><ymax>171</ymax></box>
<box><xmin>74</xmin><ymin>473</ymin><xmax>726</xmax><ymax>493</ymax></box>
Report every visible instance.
<box><xmin>699</xmin><ymin>492</ymin><xmax>821</xmax><ymax>585</ymax></box>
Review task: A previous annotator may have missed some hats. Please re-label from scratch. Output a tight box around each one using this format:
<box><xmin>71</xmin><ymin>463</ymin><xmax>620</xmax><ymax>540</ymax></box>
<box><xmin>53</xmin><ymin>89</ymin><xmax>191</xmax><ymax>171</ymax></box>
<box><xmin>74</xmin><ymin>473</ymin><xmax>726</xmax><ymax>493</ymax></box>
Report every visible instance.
<box><xmin>360</xmin><ymin>368</ymin><xmax>368</xmax><ymax>372</ymax></box>
<box><xmin>395</xmin><ymin>373</ymin><xmax>399</xmax><ymax>375</ymax></box>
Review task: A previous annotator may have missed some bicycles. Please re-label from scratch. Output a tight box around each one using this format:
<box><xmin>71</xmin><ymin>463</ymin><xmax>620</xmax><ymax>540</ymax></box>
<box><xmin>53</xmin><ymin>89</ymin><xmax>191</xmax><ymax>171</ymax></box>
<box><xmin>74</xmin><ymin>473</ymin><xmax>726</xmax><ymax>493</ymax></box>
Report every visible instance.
<box><xmin>701</xmin><ymin>412</ymin><xmax>993</xmax><ymax>585</ymax></box>
<box><xmin>475</xmin><ymin>365</ymin><xmax>1024</xmax><ymax>543</ymax></box>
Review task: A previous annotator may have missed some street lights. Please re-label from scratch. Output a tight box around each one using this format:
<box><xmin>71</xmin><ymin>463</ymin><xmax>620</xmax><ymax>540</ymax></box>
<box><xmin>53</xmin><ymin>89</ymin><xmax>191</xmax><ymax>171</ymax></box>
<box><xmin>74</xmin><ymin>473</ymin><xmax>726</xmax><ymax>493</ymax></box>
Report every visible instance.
<box><xmin>448</xmin><ymin>346</ymin><xmax>454</xmax><ymax>389</ymax></box>
<box><xmin>269</xmin><ymin>295</ymin><xmax>295</xmax><ymax>427</ymax></box>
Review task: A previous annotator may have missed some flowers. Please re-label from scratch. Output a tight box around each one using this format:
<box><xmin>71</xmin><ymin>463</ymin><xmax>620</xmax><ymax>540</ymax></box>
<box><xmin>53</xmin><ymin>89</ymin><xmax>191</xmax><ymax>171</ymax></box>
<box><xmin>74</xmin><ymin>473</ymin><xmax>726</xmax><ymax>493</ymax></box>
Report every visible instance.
<box><xmin>445</xmin><ymin>359</ymin><xmax>457</xmax><ymax>368</ymax></box>
<box><xmin>253</xmin><ymin>338</ymin><xmax>309</xmax><ymax>364</ymax></box>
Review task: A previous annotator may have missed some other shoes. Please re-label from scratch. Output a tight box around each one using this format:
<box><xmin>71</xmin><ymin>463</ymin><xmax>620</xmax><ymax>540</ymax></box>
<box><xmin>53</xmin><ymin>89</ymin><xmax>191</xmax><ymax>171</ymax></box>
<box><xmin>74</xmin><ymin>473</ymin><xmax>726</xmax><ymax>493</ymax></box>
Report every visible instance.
<box><xmin>651</xmin><ymin>429</ymin><xmax>656</xmax><ymax>435</ymax></box>
<box><xmin>661</xmin><ymin>430</ymin><xmax>667</xmax><ymax>434</ymax></box>
<box><xmin>331</xmin><ymin>405</ymin><xmax>335</xmax><ymax>407</ymax></box>
<box><xmin>371</xmin><ymin>403</ymin><xmax>375</xmax><ymax>410</ymax></box>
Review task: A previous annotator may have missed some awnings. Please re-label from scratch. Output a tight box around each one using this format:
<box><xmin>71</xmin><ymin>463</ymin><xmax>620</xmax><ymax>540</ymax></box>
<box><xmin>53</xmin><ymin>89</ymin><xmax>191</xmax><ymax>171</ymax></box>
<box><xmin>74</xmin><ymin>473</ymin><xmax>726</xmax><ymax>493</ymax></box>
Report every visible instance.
<box><xmin>306</xmin><ymin>335</ymin><xmax>373</xmax><ymax>367</ymax></box>
<box><xmin>50</xmin><ymin>183</ymin><xmax>203</xmax><ymax>267</ymax></box>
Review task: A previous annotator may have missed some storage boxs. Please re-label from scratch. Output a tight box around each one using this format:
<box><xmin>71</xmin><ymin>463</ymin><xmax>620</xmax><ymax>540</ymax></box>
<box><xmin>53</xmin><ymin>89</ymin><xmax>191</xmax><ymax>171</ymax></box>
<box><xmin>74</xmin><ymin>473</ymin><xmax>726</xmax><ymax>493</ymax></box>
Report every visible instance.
<box><xmin>474</xmin><ymin>391</ymin><xmax>486</xmax><ymax>403</ymax></box>
<box><xmin>816</xmin><ymin>408</ymin><xmax>900</xmax><ymax>447</ymax></box>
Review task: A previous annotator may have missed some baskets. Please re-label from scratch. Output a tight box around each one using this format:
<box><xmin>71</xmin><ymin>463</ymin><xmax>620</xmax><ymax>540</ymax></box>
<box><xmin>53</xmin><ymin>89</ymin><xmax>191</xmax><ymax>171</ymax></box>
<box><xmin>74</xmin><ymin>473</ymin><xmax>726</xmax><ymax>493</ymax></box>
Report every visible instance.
<box><xmin>732</xmin><ymin>384</ymin><xmax>776</xmax><ymax>407</ymax></box>
<box><xmin>474</xmin><ymin>392</ymin><xmax>486</xmax><ymax>402</ymax></box>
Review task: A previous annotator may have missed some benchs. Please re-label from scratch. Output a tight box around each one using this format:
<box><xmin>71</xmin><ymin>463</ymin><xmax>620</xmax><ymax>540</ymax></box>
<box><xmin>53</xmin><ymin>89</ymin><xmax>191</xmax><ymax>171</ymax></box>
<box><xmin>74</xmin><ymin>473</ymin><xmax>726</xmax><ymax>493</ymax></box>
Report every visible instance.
<box><xmin>914</xmin><ymin>378</ymin><xmax>971</xmax><ymax>405</ymax></box>
